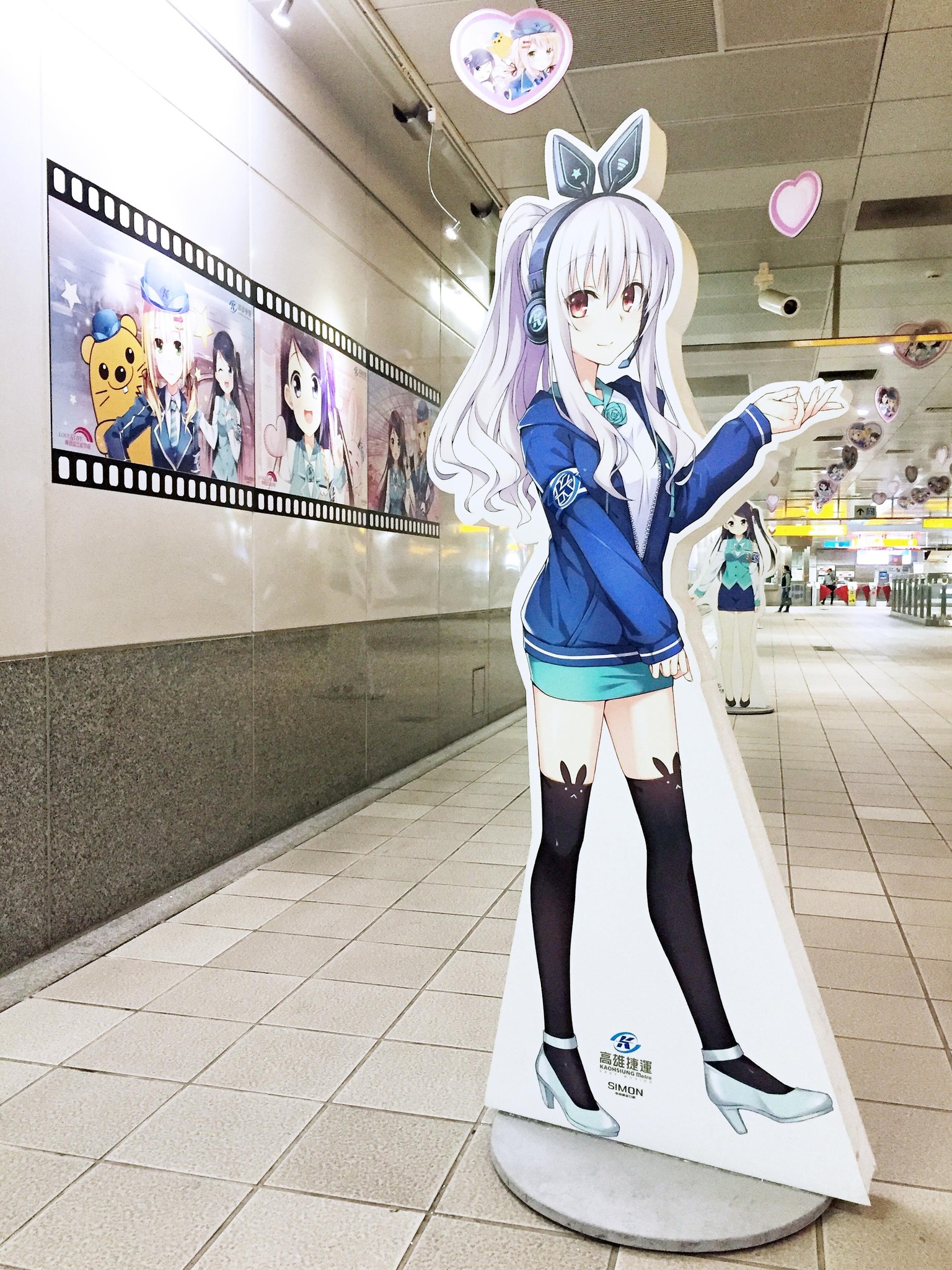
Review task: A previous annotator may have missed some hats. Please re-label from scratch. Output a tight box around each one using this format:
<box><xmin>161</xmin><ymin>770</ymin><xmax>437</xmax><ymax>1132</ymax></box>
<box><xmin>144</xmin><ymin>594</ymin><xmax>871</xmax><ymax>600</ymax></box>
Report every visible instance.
<box><xmin>827</xmin><ymin>568</ymin><xmax>832</xmax><ymax>571</ymax></box>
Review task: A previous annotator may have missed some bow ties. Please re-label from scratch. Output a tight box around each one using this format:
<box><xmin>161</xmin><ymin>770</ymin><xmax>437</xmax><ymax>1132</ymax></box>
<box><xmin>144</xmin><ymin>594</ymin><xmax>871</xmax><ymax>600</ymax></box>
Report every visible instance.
<box><xmin>827</xmin><ymin>574</ymin><xmax>830</xmax><ymax>575</ymax></box>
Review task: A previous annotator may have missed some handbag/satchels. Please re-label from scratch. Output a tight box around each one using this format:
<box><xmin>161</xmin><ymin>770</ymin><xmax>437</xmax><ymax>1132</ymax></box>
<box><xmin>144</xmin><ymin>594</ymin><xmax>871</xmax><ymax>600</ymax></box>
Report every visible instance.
<box><xmin>781</xmin><ymin>594</ymin><xmax>792</xmax><ymax>606</ymax></box>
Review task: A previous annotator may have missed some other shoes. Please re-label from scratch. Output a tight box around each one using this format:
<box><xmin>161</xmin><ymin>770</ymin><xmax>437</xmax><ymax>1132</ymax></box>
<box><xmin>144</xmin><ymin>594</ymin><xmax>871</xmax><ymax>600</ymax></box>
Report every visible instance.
<box><xmin>821</xmin><ymin>601</ymin><xmax>824</xmax><ymax>605</ymax></box>
<box><xmin>775</xmin><ymin>610</ymin><xmax>781</xmax><ymax>613</ymax></box>
<box><xmin>783</xmin><ymin>611</ymin><xmax>789</xmax><ymax>613</ymax></box>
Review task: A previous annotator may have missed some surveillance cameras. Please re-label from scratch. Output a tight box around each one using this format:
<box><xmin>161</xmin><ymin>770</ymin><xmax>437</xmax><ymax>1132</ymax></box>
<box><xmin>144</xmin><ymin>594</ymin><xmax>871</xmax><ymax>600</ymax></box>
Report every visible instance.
<box><xmin>758</xmin><ymin>288</ymin><xmax>800</xmax><ymax>318</ymax></box>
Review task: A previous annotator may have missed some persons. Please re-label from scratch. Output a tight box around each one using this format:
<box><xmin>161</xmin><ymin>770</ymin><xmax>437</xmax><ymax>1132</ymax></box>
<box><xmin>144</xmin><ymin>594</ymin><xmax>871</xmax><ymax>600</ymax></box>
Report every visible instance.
<box><xmin>776</xmin><ymin>565</ymin><xmax>792</xmax><ymax>612</ymax></box>
<box><xmin>821</xmin><ymin>568</ymin><xmax>839</xmax><ymax>605</ymax></box>
